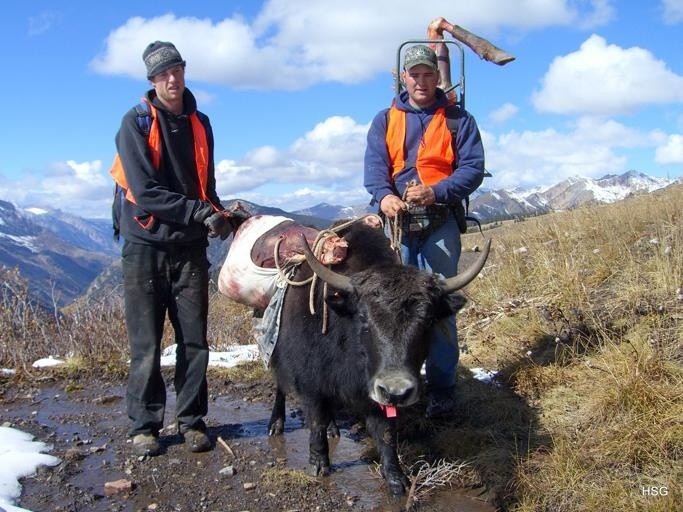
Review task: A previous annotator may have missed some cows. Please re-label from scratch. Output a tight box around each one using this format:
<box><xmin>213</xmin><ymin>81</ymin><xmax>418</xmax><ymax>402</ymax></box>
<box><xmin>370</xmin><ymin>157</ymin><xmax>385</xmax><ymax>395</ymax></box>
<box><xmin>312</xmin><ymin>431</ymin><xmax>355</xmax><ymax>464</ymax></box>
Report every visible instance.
<box><xmin>252</xmin><ymin>218</ymin><xmax>492</xmax><ymax>503</ymax></box>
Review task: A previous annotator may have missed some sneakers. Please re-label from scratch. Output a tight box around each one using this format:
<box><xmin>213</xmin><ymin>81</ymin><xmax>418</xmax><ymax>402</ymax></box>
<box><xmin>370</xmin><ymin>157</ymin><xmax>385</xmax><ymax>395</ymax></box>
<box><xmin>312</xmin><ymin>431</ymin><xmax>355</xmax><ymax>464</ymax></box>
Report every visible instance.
<box><xmin>426</xmin><ymin>391</ymin><xmax>458</xmax><ymax>420</ymax></box>
<box><xmin>184</xmin><ymin>427</ymin><xmax>211</xmax><ymax>452</ymax></box>
<box><xmin>131</xmin><ymin>431</ymin><xmax>161</xmax><ymax>456</ymax></box>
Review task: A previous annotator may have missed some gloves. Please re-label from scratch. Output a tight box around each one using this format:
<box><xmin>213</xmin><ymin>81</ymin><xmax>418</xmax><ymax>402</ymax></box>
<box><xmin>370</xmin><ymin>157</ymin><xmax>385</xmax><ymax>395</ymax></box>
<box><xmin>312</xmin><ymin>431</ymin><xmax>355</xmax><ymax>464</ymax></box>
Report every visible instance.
<box><xmin>193</xmin><ymin>200</ymin><xmax>252</xmax><ymax>240</ymax></box>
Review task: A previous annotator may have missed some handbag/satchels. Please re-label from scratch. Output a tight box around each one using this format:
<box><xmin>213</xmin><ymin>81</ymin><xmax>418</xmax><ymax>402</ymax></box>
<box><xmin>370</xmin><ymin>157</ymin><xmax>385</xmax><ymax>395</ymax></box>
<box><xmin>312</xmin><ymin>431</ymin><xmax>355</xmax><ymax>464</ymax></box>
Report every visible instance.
<box><xmin>390</xmin><ymin>203</ymin><xmax>449</xmax><ymax>242</ymax></box>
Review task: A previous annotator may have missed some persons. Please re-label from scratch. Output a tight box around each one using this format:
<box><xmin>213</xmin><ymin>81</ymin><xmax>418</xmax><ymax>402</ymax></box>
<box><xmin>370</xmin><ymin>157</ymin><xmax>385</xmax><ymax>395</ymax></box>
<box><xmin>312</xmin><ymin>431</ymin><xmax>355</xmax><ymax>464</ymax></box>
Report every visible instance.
<box><xmin>364</xmin><ymin>45</ymin><xmax>484</xmax><ymax>420</ymax></box>
<box><xmin>112</xmin><ymin>41</ymin><xmax>230</xmax><ymax>455</ymax></box>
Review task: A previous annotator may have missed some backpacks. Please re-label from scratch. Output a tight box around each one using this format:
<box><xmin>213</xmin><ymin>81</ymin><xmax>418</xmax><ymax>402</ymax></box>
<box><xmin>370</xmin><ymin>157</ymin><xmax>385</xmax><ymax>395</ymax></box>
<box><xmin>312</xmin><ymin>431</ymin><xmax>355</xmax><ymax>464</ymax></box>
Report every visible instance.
<box><xmin>445</xmin><ymin>104</ymin><xmax>470</xmax><ymax>234</ymax></box>
<box><xmin>111</xmin><ymin>102</ymin><xmax>151</xmax><ymax>242</ymax></box>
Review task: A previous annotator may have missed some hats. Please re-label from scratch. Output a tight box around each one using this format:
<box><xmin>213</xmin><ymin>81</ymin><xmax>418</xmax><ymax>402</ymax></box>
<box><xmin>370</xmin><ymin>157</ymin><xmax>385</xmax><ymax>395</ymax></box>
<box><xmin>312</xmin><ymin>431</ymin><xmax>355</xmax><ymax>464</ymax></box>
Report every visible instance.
<box><xmin>403</xmin><ymin>44</ymin><xmax>438</xmax><ymax>72</ymax></box>
<box><xmin>142</xmin><ymin>40</ymin><xmax>187</xmax><ymax>80</ymax></box>
